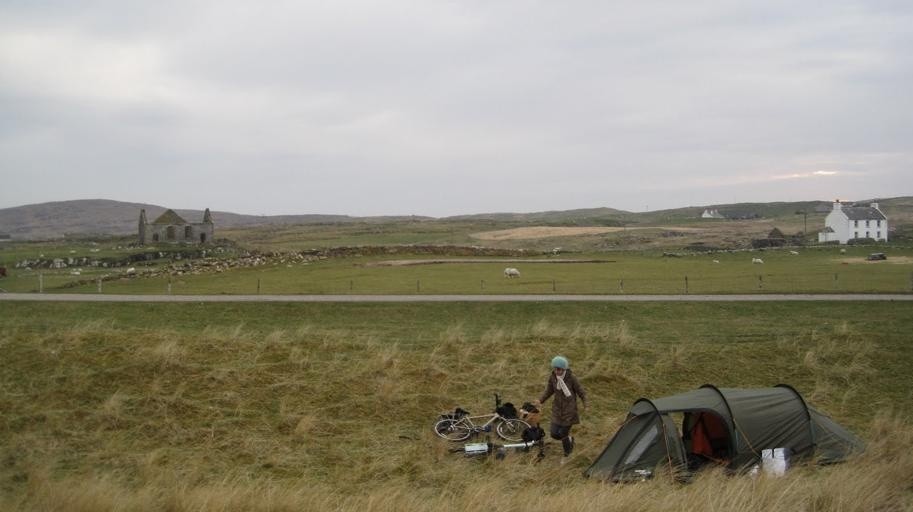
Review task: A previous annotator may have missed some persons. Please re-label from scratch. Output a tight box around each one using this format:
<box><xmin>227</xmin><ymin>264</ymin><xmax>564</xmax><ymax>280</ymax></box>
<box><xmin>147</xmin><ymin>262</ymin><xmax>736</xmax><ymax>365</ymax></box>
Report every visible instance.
<box><xmin>529</xmin><ymin>353</ymin><xmax>591</xmax><ymax>465</ymax></box>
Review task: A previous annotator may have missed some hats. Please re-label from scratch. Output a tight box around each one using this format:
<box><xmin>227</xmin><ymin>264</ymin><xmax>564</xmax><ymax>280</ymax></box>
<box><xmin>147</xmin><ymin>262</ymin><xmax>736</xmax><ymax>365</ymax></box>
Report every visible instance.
<box><xmin>550</xmin><ymin>356</ymin><xmax>569</xmax><ymax>371</ymax></box>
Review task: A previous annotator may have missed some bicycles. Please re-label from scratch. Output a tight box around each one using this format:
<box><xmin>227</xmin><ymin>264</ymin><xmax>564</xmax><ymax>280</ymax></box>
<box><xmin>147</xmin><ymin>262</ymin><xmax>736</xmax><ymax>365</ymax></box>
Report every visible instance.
<box><xmin>447</xmin><ymin>419</ymin><xmax>554</xmax><ymax>466</ymax></box>
<box><xmin>434</xmin><ymin>389</ymin><xmax>531</xmax><ymax>444</ymax></box>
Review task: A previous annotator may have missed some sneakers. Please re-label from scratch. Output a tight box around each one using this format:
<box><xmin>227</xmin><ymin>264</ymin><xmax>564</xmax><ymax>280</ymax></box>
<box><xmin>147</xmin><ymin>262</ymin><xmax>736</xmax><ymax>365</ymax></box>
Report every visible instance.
<box><xmin>559</xmin><ymin>436</ymin><xmax>577</xmax><ymax>465</ymax></box>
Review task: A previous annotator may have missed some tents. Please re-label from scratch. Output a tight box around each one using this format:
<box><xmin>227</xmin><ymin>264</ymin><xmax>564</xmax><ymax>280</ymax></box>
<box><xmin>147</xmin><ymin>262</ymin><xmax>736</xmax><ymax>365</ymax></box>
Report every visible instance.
<box><xmin>581</xmin><ymin>381</ymin><xmax>869</xmax><ymax>487</ymax></box>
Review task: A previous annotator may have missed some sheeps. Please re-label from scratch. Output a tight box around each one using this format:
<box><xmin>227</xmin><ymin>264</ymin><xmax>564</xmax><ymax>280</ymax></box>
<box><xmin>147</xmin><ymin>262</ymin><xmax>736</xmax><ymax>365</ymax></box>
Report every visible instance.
<box><xmin>840</xmin><ymin>249</ymin><xmax>846</xmax><ymax>255</ymax></box>
<box><xmin>790</xmin><ymin>250</ymin><xmax>799</xmax><ymax>256</ymax></box>
<box><xmin>752</xmin><ymin>258</ymin><xmax>764</xmax><ymax>264</ymax></box>
<box><xmin>713</xmin><ymin>259</ymin><xmax>720</xmax><ymax>264</ymax></box>
<box><xmin>503</xmin><ymin>267</ymin><xmax>521</xmax><ymax>279</ymax></box>
